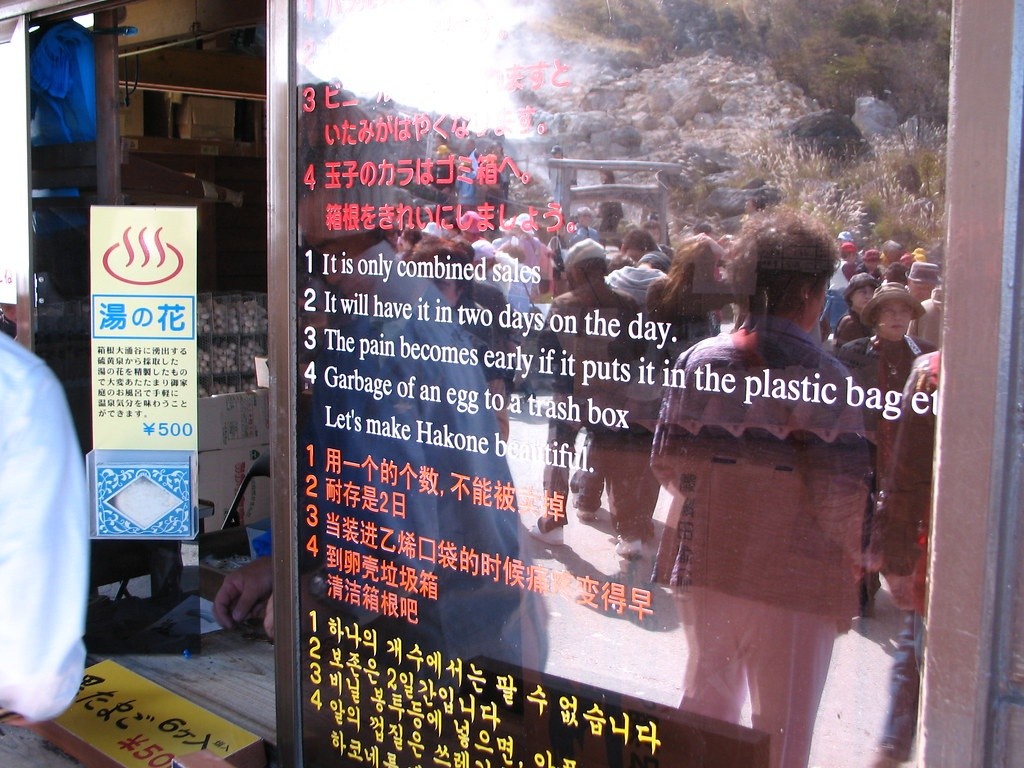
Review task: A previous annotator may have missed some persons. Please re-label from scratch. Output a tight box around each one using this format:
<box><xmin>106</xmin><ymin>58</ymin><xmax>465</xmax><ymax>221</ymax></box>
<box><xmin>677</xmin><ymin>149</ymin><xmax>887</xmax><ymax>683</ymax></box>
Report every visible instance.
<box><xmin>297</xmin><ymin>122</ymin><xmax>944</xmax><ymax>768</ymax></box>
<box><xmin>0</xmin><ymin>333</ymin><xmax>89</xmax><ymax>722</ymax></box>
<box><xmin>213</xmin><ymin>560</ymin><xmax>273</xmax><ymax>641</ymax></box>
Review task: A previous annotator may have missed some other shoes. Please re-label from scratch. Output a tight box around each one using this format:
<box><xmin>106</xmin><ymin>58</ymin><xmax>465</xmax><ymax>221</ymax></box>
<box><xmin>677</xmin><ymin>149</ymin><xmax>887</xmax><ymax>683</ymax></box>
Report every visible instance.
<box><xmin>528</xmin><ymin>524</ymin><xmax>564</xmax><ymax>545</ymax></box>
<box><xmin>616</xmin><ymin>536</ymin><xmax>641</xmax><ymax>556</ymax></box>
<box><xmin>577</xmin><ymin>508</ymin><xmax>597</xmax><ymax>521</ymax></box>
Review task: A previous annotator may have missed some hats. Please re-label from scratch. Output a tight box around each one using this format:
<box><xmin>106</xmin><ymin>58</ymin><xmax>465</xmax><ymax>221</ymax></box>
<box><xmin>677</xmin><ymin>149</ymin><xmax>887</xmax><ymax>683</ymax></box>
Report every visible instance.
<box><xmin>638</xmin><ymin>251</ymin><xmax>671</xmax><ymax>271</ymax></box>
<box><xmin>861</xmin><ymin>250</ymin><xmax>882</xmax><ymax>262</ymax></box>
<box><xmin>840</xmin><ymin>243</ymin><xmax>857</xmax><ymax>253</ymax></box>
<box><xmin>563</xmin><ymin>238</ymin><xmax>606</xmax><ymax>269</ymax></box>
<box><xmin>843</xmin><ymin>273</ymin><xmax>879</xmax><ymax>304</ymax></box>
<box><xmin>905</xmin><ymin>262</ymin><xmax>942</xmax><ymax>284</ymax></box>
<box><xmin>456</xmin><ymin>211</ymin><xmax>481</xmax><ymax>233</ymax></box>
<box><xmin>860</xmin><ymin>282</ymin><xmax>926</xmax><ymax>327</ymax></box>
<box><xmin>913</xmin><ymin>247</ymin><xmax>927</xmax><ymax>262</ymax></box>
<box><xmin>838</xmin><ymin>231</ymin><xmax>854</xmax><ymax>242</ymax></box>
